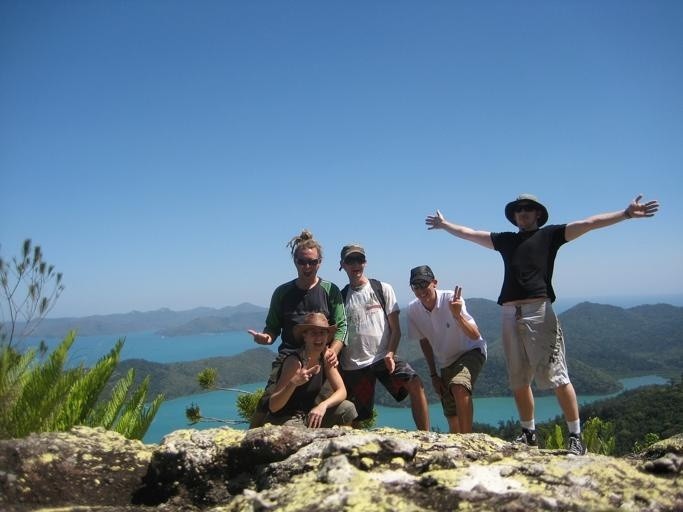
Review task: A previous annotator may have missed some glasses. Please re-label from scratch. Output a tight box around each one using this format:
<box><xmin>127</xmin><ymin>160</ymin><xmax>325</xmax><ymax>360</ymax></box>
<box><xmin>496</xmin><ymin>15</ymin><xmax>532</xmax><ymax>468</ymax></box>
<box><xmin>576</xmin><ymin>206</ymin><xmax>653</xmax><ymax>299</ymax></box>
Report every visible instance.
<box><xmin>411</xmin><ymin>280</ymin><xmax>431</xmax><ymax>289</ymax></box>
<box><xmin>513</xmin><ymin>204</ymin><xmax>538</xmax><ymax>212</ymax></box>
<box><xmin>344</xmin><ymin>256</ymin><xmax>365</xmax><ymax>265</ymax></box>
<box><xmin>294</xmin><ymin>256</ymin><xmax>321</xmax><ymax>265</ymax></box>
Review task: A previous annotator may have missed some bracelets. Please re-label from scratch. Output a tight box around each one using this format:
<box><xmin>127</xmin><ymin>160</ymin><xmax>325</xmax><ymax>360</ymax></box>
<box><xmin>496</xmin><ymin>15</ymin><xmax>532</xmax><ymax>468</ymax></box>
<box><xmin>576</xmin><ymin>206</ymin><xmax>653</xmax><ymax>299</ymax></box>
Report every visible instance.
<box><xmin>623</xmin><ymin>208</ymin><xmax>633</xmax><ymax>220</ymax></box>
<box><xmin>430</xmin><ymin>373</ymin><xmax>436</xmax><ymax>378</ymax></box>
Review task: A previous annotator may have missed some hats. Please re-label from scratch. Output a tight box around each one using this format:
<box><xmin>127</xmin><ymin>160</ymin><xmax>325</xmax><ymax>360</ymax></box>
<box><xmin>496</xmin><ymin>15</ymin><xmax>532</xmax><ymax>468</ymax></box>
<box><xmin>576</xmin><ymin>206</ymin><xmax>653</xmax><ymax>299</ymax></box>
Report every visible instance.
<box><xmin>292</xmin><ymin>312</ymin><xmax>337</xmax><ymax>345</ymax></box>
<box><xmin>504</xmin><ymin>194</ymin><xmax>548</xmax><ymax>227</ymax></box>
<box><xmin>341</xmin><ymin>244</ymin><xmax>366</xmax><ymax>263</ymax></box>
<box><xmin>409</xmin><ymin>264</ymin><xmax>435</xmax><ymax>285</ymax></box>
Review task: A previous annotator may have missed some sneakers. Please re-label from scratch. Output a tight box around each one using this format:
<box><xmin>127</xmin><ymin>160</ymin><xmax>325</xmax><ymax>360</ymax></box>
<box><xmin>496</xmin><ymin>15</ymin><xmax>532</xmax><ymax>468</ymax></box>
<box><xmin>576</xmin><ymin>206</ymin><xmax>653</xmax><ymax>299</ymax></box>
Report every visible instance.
<box><xmin>566</xmin><ymin>432</ymin><xmax>589</xmax><ymax>459</ymax></box>
<box><xmin>515</xmin><ymin>426</ymin><xmax>539</xmax><ymax>450</ymax></box>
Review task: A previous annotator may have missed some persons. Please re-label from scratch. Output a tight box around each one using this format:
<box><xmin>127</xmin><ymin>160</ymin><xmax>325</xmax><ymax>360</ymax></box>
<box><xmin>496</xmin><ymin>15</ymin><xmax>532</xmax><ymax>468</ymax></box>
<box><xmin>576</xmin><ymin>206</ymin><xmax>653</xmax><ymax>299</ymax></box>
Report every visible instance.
<box><xmin>406</xmin><ymin>265</ymin><xmax>488</xmax><ymax>434</ymax></box>
<box><xmin>339</xmin><ymin>243</ymin><xmax>430</xmax><ymax>432</ymax></box>
<box><xmin>424</xmin><ymin>192</ymin><xmax>661</xmax><ymax>458</ymax></box>
<box><xmin>265</xmin><ymin>312</ymin><xmax>359</xmax><ymax>428</ymax></box>
<box><xmin>246</xmin><ymin>230</ymin><xmax>349</xmax><ymax>428</ymax></box>
